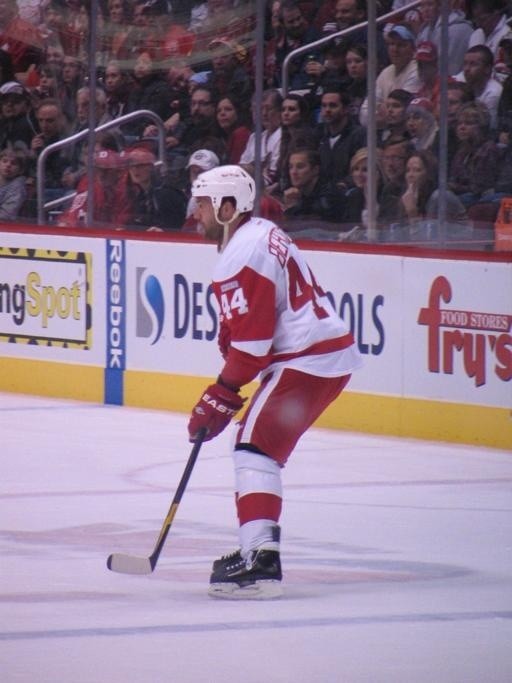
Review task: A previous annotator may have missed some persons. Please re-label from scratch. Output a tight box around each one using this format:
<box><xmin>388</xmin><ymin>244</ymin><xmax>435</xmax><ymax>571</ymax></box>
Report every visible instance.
<box><xmin>1</xmin><ymin>0</ymin><xmax>512</xmax><ymax>252</ymax></box>
<box><xmin>188</xmin><ymin>165</ymin><xmax>365</xmax><ymax>600</ymax></box>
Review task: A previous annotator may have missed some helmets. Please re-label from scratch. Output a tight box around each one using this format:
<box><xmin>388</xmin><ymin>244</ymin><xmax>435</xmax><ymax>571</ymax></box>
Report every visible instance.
<box><xmin>191</xmin><ymin>165</ymin><xmax>256</xmax><ymax>214</ymax></box>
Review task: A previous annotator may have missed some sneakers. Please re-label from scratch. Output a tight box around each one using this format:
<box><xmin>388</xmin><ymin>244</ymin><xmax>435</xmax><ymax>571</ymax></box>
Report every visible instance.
<box><xmin>209</xmin><ymin>547</ymin><xmax>282</xmax><ymax>584</ymax></box>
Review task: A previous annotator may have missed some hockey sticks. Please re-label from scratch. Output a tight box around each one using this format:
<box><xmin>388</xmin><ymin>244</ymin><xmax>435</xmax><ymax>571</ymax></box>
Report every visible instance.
<box><xmin>107</xmin><ymin>428</ymin><xmax>208</xmax><ymax>575</ymax></box>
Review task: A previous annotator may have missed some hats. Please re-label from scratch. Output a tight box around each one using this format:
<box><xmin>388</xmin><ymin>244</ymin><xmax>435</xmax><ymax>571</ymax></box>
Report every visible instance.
<box><xmin>2</xmin><ymin>81</ymin><xmax>26</xmax><ymax>99</ymax></box>
<box><xmin>184</xmin><ymin>148</ymin><xmax>219</xmax><ymax>172</ymax></box>
<box><xmin>405</xmin><ymin>96</ymin><xmax>435</xmax><ymax>121</ymax></box>
<box><xmin>95</xmin><ymin>149</ymin><xmax>119</xmax><ymax>168</ymax></box>
<box><xmin>413</xmin><ymin>40</ymin><xmax>437</xmax><ymax>61</ymax></box>
<box><xmin>128</xmin><ymin>150</ymin><xmax>157</xmax><ymax>167</ymax></box>
<box><xmin>389</xmin><ymin>21</ymin><xmax>416</xmax><ymax>41</ymax></box>
<box><xmin>208</xmin><ymin>36</ymin><xmax>238</xmax><ymax>53</ymax></box>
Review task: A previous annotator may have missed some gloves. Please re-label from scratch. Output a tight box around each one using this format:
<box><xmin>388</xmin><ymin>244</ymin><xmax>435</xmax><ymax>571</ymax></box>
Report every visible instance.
<box><xmin>188</xmin><ymin>385</ymin><xmax>244</xmax><ymax>443</ymax></box>
